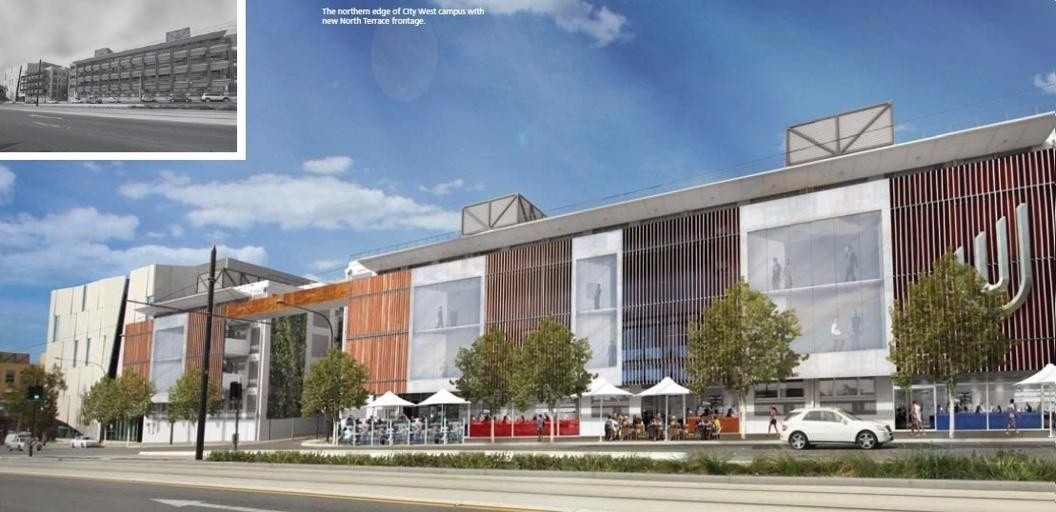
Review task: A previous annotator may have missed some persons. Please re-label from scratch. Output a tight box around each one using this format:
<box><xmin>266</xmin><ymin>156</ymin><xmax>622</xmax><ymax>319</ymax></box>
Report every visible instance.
<box><xmin>338</xmin><ymin>415</ymin><xmax>461</xmax><ymax>445</ymax></box>
<box><xmin>449</xmin><ymin>307</ymin><xmax>457</xmax><ymax>327</ymax></box>
<box><xmin>592</xmin><ymin>283</ymin><xmax>601</xmax><ymax>310</ymax></box>
<box><xmin>605</xmin><ymin>340</ymin><xmax>615</xmax><ymax>369</ymax></box>
<box><xmin>830</xmin><ymin>315</ymin><xmax>843</xmax><ymax>352</ymax></box>
<box><xmin>770</xmin><ymin>256</ymin><xmax>780</xmax><ymax>291</ymax></box>
<box><xmin>844</xmin><ymin>244</ymin><xmax>857</xmax><ymax>282</ymax></box>
<box><xmin>469</xmin><ymin>413</ymin><xmax>552</xmax><ymax>422</ymax></box>
<box><xmin>782</xmin><ymin>256</ymin><xmax>792</xmax><ymax>288</ymax></box>
<box><xmin>433</xmin><ymin>307</ymin><xmax>444</xmax><ymax>328</ymax></box>
<box><xmin>605</xmin><ymin>402</ymin><xmax>733</xmax><ymax>440</ymax></box>
<box><xmin>765</xmin><ymin>405</ymin><xmax>779</xmax><ymax>438</ymax></box>
<box><xmin>909</xmin><ymin>398</ymin><xmax>1032</xmax><ymax>436</ymax></box>
<box><xmin>536</xmin><ymin>414</ymin><xmax>543</xmax><ymax>444</ymax></box>
<box><xmin>438</xmin><ymin>360</ymin><xmax>448</xmax><ymax>379</ymax></box>
<box><xmin>846</xmin><ymin>310</ymin><xmax>864</xmax><ymax>350</ymax></box>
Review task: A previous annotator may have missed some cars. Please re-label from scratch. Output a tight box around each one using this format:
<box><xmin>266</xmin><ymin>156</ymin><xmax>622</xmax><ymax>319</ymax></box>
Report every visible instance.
<box><xmin>141</xmin><ymin>94</ymin><xmax>157</xmax><ymax>102</ymax></box>
<box><xmin>167</xmin><ymin>94</ymin><xmax>192</xmax><ymax>102</ymax></box>
<box><xmin>71</xmin><ymin>436</ymin><xmax>100</xmax><ymax>448</ymax></box>
<box><xmin>6</xmin><ymin>437</ymin><xmax>31</xmax><ymax>451</ymax></box>
<box><xmin>25</xmin><ymin>95</ymin><xmax>102</xmax><ymax>104</ymax></box>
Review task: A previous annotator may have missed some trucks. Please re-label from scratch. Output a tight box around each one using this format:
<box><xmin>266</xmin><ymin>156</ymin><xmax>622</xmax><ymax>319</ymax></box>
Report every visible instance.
<box><xmin>4</xmin><ymin>432</ymin><xmax>33</xmax><ymax>446</ymax></box>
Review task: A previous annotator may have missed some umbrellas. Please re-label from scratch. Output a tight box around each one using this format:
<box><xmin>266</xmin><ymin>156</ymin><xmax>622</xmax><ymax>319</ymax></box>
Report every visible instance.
<box><xmin>635</xmin><ymin>376</ymin><xmax>692</xmax><ymax>441</ymax></box>
<box><xmin>361</xmin><ymin>389</ymin><xmax>414</xmax><ymax>419</ymax></box>
<box><xmin>570</xmin><ymin>382</ymin><xmax>634</xmax><ymax>442</ymax></box>
<box><xmin>415</xmin><ymin>389</ymin><xmax>471</xmax><ymax>423</ymax></box>
<box><xmin>1013</xmin><ymin>362</ymin><xmax>1056</xmax><ymax>439</ymax></box>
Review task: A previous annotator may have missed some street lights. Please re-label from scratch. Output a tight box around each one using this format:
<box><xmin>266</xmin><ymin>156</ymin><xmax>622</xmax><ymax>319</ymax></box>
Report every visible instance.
<box><xmin>53</xmin><ymin>355</ymin><xmax>107</xmax><ymax>381</ymax></box>
<box><xmin>275</xmin><ymin>299</ymin><xmax>333</xmax><ymax>443</ymax></box>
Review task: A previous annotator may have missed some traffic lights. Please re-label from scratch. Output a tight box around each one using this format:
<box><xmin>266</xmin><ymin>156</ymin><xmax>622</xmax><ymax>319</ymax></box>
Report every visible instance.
<box><xmin>231</xmin><ymin>382</ymin><xmax>238</xmax><ymax>400</ymax></box>
<box><xmin>26</xmin><ymin>384</ymin><xmax>32</xmax><ymax>399</ymax></box>
<box><xmin>33</xmin><ymin>385</ymin><xmax>40</xmax><ymax>400</ymax></box>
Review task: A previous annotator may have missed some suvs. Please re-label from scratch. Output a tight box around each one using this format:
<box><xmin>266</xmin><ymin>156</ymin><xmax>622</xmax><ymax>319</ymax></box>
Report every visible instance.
<box><xmin>780</xmin><ymin>407</ymin><xmax>895</xmax><ymax>449</ymax></box>
<box><xmin>200</xmin><ymin>92</ymin><xmax>231</xmax><ymax>102</ymax></box>
